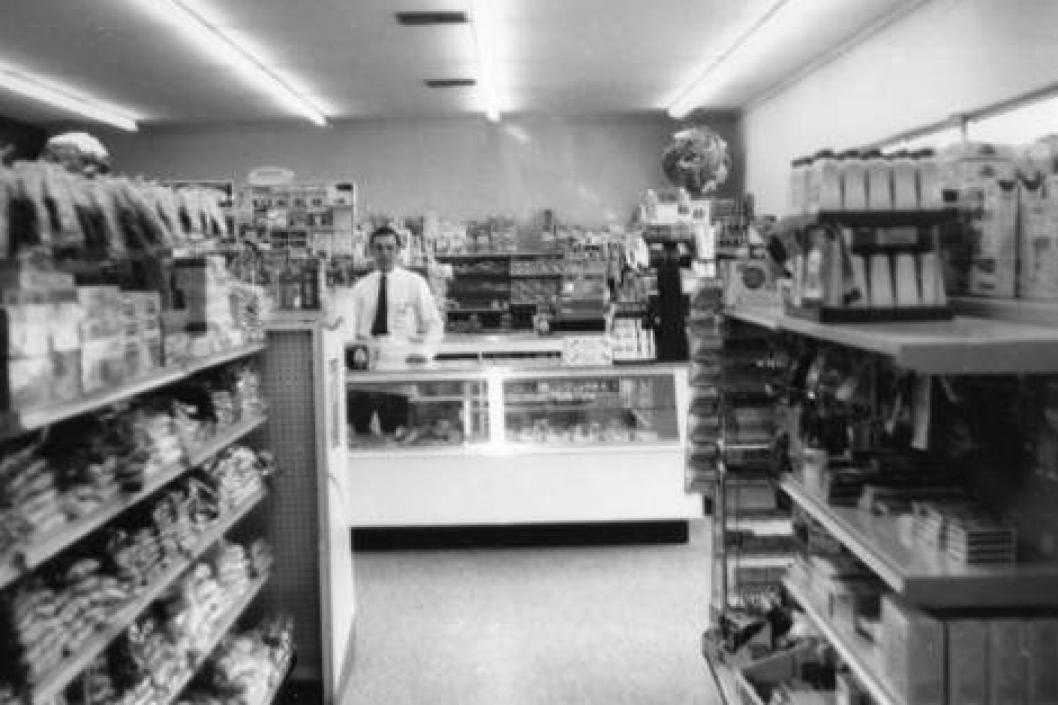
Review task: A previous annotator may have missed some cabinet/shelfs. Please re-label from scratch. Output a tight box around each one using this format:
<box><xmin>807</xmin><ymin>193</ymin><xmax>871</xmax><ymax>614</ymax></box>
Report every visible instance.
<box><xmin>702</xmin><ymin>291</ymin><xmax>1058</xmax><ymax>705</ymax></box>
<box><xmin>399</xmin><ymin>223</ymin><xmax>642</xmax><ymax>332</ymax></box>
<box><xmin>0</xmin><ymin>344</ymin><xmax>300</xmax><ymax>705</ymax></box>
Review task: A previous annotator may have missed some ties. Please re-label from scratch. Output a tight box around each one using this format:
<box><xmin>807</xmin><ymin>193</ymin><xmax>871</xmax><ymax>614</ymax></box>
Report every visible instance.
<box><xmin>371</xmin><ymin>275</ymin><xmax>388</xmax><ymax>336</ymax></box>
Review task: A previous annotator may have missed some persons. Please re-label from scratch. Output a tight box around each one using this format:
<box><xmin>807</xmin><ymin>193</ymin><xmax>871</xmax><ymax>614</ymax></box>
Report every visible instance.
<box><xmin>340</xmin><ymin>225</ymin><xmax>443</xmax><ymax>438</ymax></box>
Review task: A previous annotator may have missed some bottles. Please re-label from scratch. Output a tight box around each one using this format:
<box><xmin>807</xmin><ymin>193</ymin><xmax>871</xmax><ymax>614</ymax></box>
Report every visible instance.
<box><xmin>789</xmin><ymin>151</ymin><xmax>940</xmax><ymax>210</ymax></box>
<box><xmin>849</xmin><ymin>245</ymin><xmax>940</xmax><ymax>304</ymax></box>
